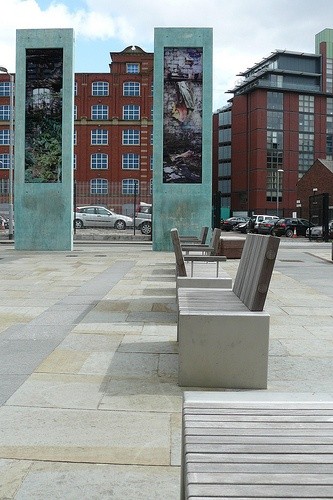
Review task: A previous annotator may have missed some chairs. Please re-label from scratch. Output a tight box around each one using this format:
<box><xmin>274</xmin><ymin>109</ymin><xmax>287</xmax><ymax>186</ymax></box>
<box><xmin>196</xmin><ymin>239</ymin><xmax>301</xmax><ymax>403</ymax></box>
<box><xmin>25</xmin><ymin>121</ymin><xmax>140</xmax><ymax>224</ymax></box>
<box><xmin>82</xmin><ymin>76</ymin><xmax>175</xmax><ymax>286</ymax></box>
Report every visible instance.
<box><xmin>171</xmin><ymin>226</ymin><xmax>221</xmax><ymax>276</ymax></box>
<box><xmin>176</xmin><ymin>232</ymin><xmax>281</xmax><ymax>388</ymax></box>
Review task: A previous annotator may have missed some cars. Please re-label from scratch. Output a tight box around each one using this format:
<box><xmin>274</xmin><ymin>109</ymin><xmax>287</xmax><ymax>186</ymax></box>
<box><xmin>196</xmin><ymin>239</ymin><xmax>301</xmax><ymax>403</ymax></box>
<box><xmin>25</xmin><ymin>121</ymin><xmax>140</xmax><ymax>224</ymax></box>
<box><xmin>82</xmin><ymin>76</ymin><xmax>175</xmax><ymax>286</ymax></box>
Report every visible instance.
<box><xmin>75</xmin><ymin>206</ymin><xmax>134</xmax><ymax>230</ymax></box>
<box><xmin>221</xmin><ymin>213</ymin><xmax>333</xmax><ymax>241</ymax></box>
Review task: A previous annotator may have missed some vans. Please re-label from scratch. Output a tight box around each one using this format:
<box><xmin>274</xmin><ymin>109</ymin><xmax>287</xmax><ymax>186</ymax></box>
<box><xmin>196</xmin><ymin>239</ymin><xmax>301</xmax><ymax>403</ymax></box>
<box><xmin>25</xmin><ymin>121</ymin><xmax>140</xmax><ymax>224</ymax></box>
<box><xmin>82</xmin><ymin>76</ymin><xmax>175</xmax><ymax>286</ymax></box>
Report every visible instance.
<box><xmin>134</xmin><ymin>202</ymin><xmax>153</xmax><ymax>234</ymax></box>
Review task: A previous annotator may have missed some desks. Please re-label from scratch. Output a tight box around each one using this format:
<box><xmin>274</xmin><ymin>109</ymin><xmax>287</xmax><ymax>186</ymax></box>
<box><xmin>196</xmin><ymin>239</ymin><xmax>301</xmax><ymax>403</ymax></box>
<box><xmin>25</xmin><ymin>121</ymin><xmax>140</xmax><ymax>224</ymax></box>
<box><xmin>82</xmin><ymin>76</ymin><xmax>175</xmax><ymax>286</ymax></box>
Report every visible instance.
<box><xmin>183</xmin><ymin>255</ymin><xmax>226</xmax><ymax>278</ymax></box>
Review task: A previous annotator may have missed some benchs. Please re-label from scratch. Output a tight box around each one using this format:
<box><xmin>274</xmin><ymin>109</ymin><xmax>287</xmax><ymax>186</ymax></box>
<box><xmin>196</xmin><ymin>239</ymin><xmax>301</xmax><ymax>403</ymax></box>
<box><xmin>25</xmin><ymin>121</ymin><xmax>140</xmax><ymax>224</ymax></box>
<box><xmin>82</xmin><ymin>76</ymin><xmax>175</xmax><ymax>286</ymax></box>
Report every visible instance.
<box><xmin>179</xmin><ymin>390</ymin><xmax>333</xmax><ymax>499</ymax></box>
